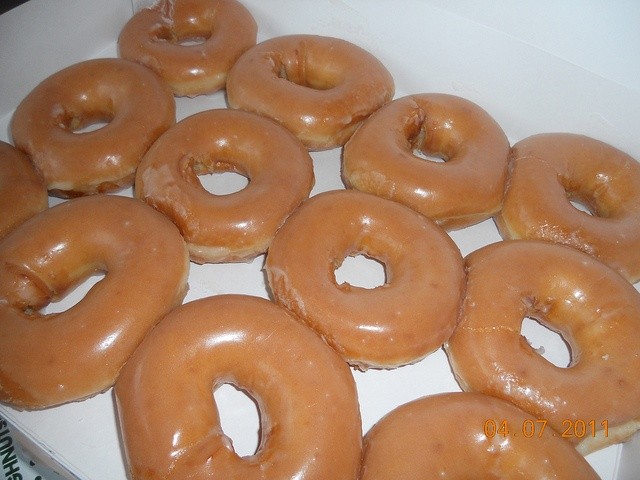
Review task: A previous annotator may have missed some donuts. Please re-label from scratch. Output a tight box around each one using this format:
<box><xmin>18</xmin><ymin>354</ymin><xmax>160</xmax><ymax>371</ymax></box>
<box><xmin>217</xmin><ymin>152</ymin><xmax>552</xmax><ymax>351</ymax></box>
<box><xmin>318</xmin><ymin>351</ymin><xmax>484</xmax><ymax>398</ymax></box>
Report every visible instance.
<box><xmin>0</xmin><ymin>194</ymin><xmax>190</xmax><ymax>411</ymax></box>
<box><xmin>0</xmin><ymin>140</ymin><xmax>49</xmax><ymax>239</ymax></box>
<box><xmin>497</xmin><ymin>132</ymin><xmax>639</xmax><ymax>284</ymax></box>
<box><xmin>262</xmin><ymin>188</ymin><xmax>466</xmax><ymax>371</ymax></box>
<box><xmin>11</xmin><ymin>58</ymin><xmax>177</xmax><ymax>199</ymax></box>
<box><xmin>342</xmin><ymin>94</ymin><xmax>511</xmax><ymax>232</ymax></box>
<box><xmin>443</xmin><ymin>238</ymin><xmax>640</xmax><ymax>456</ymax></box>
<box><xmin>133</xmin><ymin>108</ymin><xmax>315</xmax><ymax>264</ymax></box>
<box><xmin>113</xmin><ymin>294</ymin><xmax>361</xmax><ymax>479</ymax></box>
<box><xmin>360</xmin><ymin>393</ymin><xmax>600</xmax><ymax>480</ymax></box>
<box><xmin>225</xmin><ymin>34</ymin><xmax>395</xmax><ymax>151</ymax></box>
<box><xmin>117</xmin><ymin>0</ymin><xmax>258</xmax><ymax>100</ymax></box>
